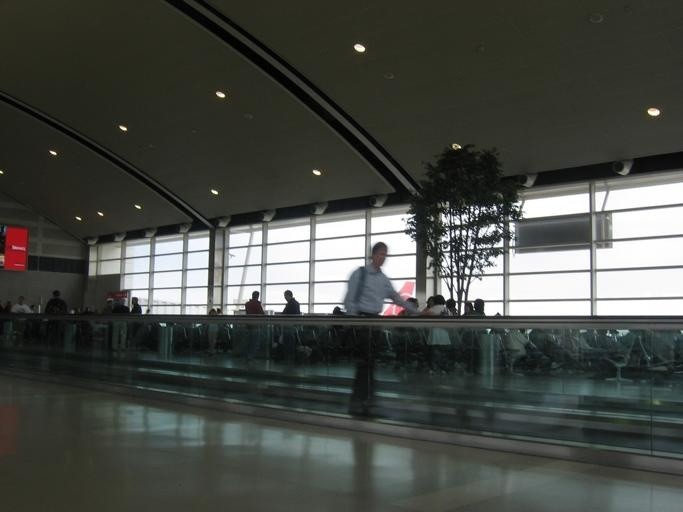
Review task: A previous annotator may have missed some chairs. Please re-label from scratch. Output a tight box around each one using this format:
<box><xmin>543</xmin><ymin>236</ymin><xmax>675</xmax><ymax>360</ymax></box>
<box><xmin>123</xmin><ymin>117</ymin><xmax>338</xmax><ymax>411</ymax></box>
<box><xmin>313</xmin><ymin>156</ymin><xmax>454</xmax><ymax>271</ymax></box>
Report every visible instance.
<box><xmin>174</xmin><ymin>312</ymin><xmax>683</xmax><ymax>385</ymax></box>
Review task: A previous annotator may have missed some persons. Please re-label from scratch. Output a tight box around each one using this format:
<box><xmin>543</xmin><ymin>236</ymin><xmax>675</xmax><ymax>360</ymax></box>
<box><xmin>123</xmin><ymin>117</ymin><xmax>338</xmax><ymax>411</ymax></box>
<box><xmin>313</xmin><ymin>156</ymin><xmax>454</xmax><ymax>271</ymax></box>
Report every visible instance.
<box><xmin>283</xmin><ymin>290</ymin><xmax>300</xmax><ymax>314</ymax></box>
<box><xmin>392</xmin><ymin>295</ymin><xmax>555</xmax><ymax>378</ymax></box>
<box><xmin>344</xmin><ymin>242</ymin><xmax>417</xmax><ymax>416</ymax></box>
<box><xmin>0</xmin><ymin>290</ymin><xmax>141</xmax><ymax>348</ymax></box>
<box><xmin>245</xmin><ymin>291</ymin><xmax>265</xmax><ymax>315</ymax></box>
<box><xmin>208</xmin><ymin>309</ymin><xmax>218</xmax><ymax>354</ymax></box>
<box><xmin>215</xmin><ymin>308</ymin><xmax>229</xmax><ymax>352</ymax></box>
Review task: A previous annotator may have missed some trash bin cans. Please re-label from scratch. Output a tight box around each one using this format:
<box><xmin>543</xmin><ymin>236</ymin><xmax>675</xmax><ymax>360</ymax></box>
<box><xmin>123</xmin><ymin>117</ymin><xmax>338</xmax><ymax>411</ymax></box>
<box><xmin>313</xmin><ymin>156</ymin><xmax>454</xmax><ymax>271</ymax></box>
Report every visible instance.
<box><xmin>479</xmin><ymin>334</ymin><xmax>502</xmax><ymax>376</ymax></box>
<box><xmin>158</xmin><ymin>323</ymin><xmax>174</xmax><ymax>359</ymax></box>
